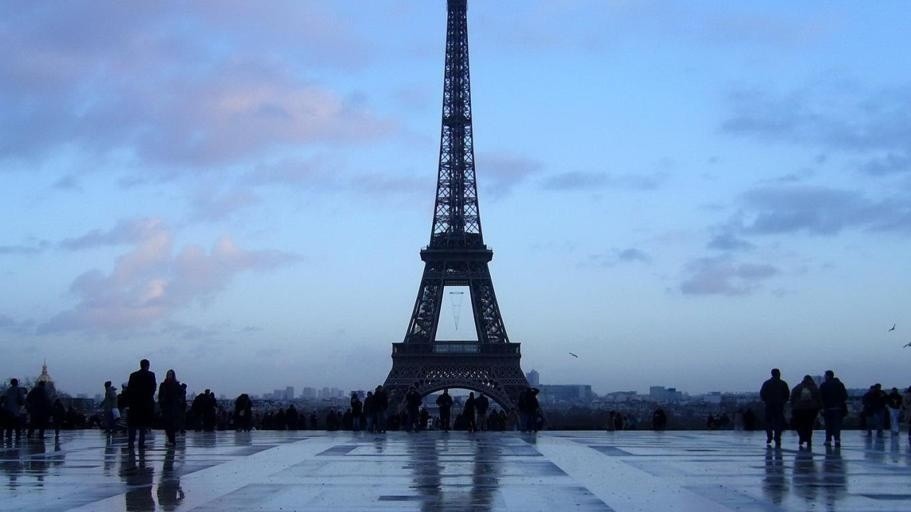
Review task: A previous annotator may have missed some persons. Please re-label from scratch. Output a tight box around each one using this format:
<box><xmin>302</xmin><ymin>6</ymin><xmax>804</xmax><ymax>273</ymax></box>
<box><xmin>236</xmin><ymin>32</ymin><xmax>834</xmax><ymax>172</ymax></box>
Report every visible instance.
<box><xmin>862</xmin><ymin>384</ymin><xmax>911</xmax><ymax>443</ymax></box>
<box><xmin>760</xmin><ymin>368</ymin><xmax>790</xmax><ymax>443</ymax></box>
<box><xmin>820</xmin><ymin>371</ymin><xmax>848</xmax><ymax>446</ymax></box>
<box><xmin>792</xmin><ymin>375</ymin><xmax>819</xmax><ymax>446</ymax></box>
<box><xmin>608</xmin><ymin>406</ymin><xmax>667</xmax><ymax>430</ymax></box>
<box><xmin>707</xmin><ymin>406</ymin><xmax>759</xmax><ymax>429</ymax></box>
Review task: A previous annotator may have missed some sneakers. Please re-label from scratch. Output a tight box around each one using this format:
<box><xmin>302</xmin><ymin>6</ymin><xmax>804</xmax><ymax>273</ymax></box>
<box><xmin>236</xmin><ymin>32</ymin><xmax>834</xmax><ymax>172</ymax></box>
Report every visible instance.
<box><xmin>765</xmin><ymin>428</ymin><xmax>902</xmax><ymax>449</ymax></box>
<box><xmin>0</xmin><ymin>429</ymin><xmax>259</xmax><ymax>450</ymax></box>
